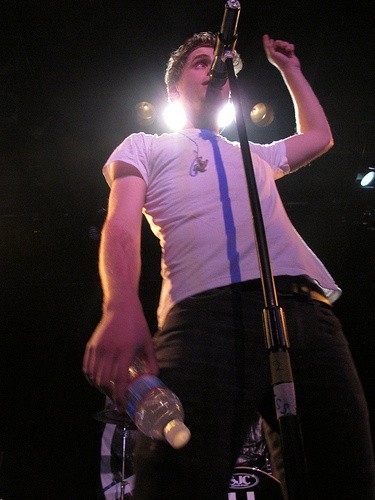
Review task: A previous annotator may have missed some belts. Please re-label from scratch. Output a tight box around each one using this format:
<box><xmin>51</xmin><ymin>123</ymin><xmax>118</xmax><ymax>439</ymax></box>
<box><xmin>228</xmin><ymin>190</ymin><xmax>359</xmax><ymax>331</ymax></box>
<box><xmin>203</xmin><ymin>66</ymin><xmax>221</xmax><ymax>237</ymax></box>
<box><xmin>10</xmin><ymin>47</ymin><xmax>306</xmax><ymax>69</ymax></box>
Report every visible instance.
<box><xmin>197</xmin><ymin>279</ymin><xmax>333</xmax><ymax>305</ymax></box>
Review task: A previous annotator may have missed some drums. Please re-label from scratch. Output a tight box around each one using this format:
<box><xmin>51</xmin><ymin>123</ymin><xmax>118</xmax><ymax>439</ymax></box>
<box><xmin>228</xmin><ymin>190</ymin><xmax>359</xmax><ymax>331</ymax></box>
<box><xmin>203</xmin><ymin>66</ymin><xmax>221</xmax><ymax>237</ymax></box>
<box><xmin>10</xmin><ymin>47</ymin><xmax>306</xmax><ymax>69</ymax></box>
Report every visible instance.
<box><xmin>227</xmin><ymin>467</ymin><xmax>282</xmax><ymax>500</ymax></box>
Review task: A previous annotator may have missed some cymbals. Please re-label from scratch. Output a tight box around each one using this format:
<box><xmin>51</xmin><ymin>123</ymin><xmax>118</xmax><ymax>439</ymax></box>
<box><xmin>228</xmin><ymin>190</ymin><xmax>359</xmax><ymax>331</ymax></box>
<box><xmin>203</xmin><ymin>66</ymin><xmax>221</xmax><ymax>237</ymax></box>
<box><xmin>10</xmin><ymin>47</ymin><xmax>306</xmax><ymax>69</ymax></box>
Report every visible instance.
<box><xmin>93</xmin><ymin>410</ymin><xmax>138</xmax><ymax>430</ymax></box>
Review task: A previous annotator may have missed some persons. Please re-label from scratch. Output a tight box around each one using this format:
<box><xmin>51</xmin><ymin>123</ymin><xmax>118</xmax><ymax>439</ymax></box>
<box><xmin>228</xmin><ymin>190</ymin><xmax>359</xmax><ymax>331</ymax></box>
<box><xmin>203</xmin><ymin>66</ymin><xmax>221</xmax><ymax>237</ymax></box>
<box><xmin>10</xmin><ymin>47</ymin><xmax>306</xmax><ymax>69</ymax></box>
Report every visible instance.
<box><xmin>82</xmin><ymin>32</ymin><xmax>375</xmax><ymax>500</ymax></box>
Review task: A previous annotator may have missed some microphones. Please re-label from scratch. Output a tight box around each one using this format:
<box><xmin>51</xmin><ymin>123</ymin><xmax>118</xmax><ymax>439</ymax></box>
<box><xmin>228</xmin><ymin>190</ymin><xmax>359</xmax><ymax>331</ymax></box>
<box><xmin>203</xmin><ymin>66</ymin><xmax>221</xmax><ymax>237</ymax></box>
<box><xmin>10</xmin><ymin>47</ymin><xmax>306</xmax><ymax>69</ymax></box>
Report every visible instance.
<box><xmin>208</xmin><ymin>0</ymin><xmax>240</xmax><ymax>88</ymax></box>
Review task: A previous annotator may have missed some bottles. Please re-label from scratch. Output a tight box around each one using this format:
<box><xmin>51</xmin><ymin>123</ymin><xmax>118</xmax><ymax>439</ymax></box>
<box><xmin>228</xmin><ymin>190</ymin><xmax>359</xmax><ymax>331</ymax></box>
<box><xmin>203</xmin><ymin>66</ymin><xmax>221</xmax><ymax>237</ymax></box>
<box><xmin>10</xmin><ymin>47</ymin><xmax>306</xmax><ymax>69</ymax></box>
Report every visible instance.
<box><xmin>110</xmin><ymin>355</ymin><xmax>190</xmax><ymax>448</ymax></box>
